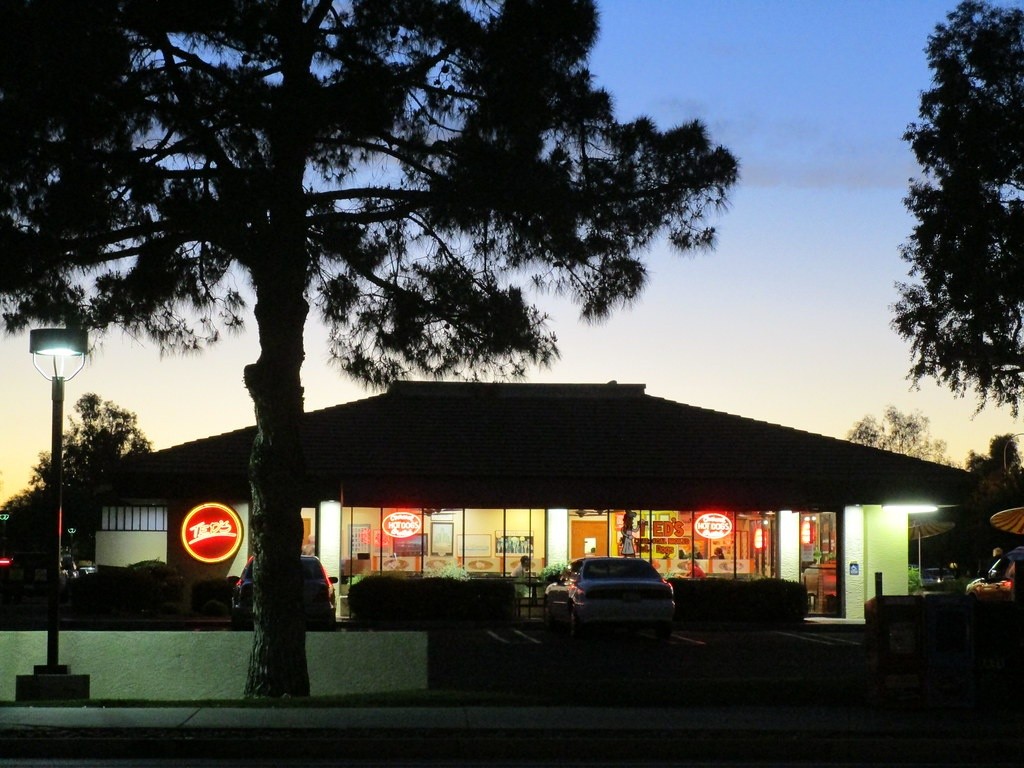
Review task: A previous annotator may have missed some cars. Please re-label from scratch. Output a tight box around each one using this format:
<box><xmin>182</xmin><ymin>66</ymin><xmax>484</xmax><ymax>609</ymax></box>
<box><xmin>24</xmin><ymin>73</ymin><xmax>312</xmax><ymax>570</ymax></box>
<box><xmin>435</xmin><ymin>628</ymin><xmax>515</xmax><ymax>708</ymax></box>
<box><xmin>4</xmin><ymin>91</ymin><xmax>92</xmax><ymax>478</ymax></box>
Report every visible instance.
<box><xmin>917</xmin><ymin>567</ymin><xmax>957</xmax><ymax>588</ymax></box>
<box><xmin>967</xmin><ymin>578</ymin><xmax>1012</xmax><ymax>600</ymax></box>
<box><xmin>543</xmin><ymin>556</ymin><xmax>676</xmax><ymax>643</ymax></box>
<box><xmin>226</xmin><ymin>556</ymin><xmax>338</xmax><ymax>633</ymax></box>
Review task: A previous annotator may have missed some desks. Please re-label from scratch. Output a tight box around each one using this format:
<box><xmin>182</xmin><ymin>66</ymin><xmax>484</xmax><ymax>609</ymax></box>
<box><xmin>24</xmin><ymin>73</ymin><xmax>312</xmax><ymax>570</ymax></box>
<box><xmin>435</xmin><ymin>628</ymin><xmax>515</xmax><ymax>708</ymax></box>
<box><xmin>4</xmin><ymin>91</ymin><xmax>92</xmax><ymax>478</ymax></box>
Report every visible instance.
<box><xmin>526</xmin><ymin>581</ymin><xmax>545</xmax><ymax>608</ymax></box>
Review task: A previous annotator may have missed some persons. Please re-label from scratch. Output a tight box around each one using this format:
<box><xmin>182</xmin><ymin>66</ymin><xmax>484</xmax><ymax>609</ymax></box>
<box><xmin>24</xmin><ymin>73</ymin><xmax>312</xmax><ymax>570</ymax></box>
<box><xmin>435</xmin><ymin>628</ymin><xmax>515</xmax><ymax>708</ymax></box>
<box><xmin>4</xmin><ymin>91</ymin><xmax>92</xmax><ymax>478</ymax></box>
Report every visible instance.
<box><xmin>662</xmin><ymin>547</ymin><xmax>725</xmax><ymax>559</ymax></box>
<box><xmin>990</xmin><ymin>547</ymin><xmax>1006</xmax><ymax>577</ymax></box>
<box><xmin>512</xmin><ymin>556</ymin><xmax>533</xmax><ymax>577</ymax></box>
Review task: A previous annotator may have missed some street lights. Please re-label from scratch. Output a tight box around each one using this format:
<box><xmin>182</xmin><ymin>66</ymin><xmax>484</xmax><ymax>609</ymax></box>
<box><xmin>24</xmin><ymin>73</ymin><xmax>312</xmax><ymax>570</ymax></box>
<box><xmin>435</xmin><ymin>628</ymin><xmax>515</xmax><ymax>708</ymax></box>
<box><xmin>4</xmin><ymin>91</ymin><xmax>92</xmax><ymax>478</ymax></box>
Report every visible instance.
<box><xmin>0</xmin><ymin>510</ymin><xmax>10</xmax><ymax>528</ymax></box>
<box><xmin>14</xmin><ymin>329</ymin><xmax>89</xmax><ymax>701</ymax></box>
<box><xmin>67</xmin><ymin>527</ymin><xmax>76</xmax><ymax>544</ymax></box>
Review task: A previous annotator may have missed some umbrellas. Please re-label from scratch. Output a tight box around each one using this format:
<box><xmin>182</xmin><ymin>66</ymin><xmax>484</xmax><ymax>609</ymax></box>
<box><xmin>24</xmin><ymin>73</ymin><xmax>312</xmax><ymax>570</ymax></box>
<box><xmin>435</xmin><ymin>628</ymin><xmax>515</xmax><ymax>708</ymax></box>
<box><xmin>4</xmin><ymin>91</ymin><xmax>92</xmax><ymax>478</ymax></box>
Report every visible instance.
<box><xmin>990</xmin><ymin>507</ymin><xmax>1024</xmax><ymax>534</ymax></box>
<box><xmin>909</xmin><ymin>519</ymin><xmax>956</xmax><ymax>580</ymax></box>
